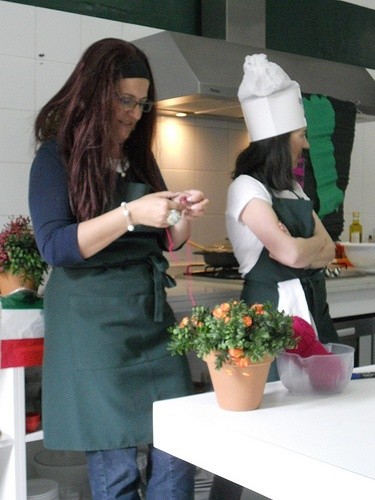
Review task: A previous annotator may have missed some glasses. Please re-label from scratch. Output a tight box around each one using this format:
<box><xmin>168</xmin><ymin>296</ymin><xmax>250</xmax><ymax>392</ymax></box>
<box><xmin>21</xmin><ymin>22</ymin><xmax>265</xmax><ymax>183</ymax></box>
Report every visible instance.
<box><xmin>114</xmin><ymin>92</ymin><xmax>155</xmax><ymax>113</ymax></box>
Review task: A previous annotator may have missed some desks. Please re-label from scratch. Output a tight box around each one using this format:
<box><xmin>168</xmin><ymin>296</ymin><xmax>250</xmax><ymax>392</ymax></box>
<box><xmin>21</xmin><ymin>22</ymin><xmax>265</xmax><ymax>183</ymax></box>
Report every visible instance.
<box><xmin>153</xmin><ymin>364</ymin><xmax>374</xmax><ymax>500</ymax></box>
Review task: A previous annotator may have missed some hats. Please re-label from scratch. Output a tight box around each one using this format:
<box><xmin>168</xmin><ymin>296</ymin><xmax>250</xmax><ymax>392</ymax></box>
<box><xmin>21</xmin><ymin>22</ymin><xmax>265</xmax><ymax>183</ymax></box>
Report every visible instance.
<box><xmin>237</xmin><ymin>54</ymin><xmax>308</xmax><ymax>143</ymax></box>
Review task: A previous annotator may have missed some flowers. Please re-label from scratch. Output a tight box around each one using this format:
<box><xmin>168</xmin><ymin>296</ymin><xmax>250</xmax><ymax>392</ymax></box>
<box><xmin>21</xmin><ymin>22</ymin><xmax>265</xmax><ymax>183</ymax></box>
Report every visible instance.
<box><xmin>0</xmin><ymin>216</ymin><xmax>50</xmax><ymax>291</ymax></box>
<box><xmin>161</xmin><ymin>298</ymin><xmax>302</xmax><ymax>372</ymax></box>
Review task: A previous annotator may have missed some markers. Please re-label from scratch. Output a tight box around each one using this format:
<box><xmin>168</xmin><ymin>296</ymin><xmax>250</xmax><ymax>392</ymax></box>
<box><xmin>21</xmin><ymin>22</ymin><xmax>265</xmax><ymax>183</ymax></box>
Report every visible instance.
<box><xmin>349</xmin><ymin>371</ymin><xmax>375</xmax><ymax>380</ymax></box>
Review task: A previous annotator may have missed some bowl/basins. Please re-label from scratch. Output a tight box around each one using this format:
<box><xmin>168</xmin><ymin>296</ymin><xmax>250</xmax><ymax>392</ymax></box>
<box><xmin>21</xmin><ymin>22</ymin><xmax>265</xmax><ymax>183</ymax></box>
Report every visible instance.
<box><xmin>274</xmin><ymin>342</ymin><xmax>355</xmax><ymax>399</ymax></box>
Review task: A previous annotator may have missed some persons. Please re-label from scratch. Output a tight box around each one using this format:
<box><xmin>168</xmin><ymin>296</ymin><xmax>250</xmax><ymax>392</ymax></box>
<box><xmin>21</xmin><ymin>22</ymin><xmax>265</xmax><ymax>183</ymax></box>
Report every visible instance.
<box><xmin>209</xmin><ymin>53</ymin><xmax>343</xmax><ymax>500</ymax></box>
<box><xmin>28</xmin><ymin>38</ymin><xmax>209</xmax><ymax>500</ymax></box>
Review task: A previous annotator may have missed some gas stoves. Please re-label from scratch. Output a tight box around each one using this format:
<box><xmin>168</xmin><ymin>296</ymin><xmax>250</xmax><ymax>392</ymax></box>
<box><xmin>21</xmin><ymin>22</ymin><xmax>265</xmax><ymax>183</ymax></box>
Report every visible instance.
<box><xmin>172</xmin><ymin>263</ymin><xmax>375</xmax><ymax>292</ymax></box>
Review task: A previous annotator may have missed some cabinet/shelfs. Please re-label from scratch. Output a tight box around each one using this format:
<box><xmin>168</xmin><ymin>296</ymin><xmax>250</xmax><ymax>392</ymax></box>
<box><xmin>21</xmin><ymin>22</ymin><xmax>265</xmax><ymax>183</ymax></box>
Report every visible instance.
<box><xmin>1</xmin><ymin>364</ymin><xmax>43</xmax><ymax>500</ymax></box>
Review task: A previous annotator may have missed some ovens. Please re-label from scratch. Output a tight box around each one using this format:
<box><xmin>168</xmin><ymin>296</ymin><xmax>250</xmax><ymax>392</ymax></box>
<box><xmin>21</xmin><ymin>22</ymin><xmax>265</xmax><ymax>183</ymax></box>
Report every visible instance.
<box><xmin>324</xmin><ymin>293</ymin><xmax>375</xmax><ymax>369</ymax></box>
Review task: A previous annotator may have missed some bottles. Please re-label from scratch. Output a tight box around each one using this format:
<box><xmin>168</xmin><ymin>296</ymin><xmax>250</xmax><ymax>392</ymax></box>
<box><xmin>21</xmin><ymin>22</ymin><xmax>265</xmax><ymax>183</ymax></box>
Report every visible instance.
<box><xmin>349</xmin><ymin>211</ymin><xmax>363</xmax><ymax>243</ymax></box>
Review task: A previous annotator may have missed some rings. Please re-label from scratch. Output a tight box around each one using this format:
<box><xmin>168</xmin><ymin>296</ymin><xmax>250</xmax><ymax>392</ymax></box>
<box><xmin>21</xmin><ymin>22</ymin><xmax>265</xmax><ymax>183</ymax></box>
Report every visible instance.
<box><xmin>166</xmin><ymin>208</ymin><xmax>181</xmax><ymax>226</ymax></box>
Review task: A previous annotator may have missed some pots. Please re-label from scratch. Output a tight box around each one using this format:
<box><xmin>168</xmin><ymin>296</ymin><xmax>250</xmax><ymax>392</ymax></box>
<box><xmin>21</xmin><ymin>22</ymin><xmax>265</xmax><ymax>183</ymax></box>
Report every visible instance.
<box><xmin>184</xmin><ymin>236</ymin><xmax>239</xmax><ymax>266</ymax></box>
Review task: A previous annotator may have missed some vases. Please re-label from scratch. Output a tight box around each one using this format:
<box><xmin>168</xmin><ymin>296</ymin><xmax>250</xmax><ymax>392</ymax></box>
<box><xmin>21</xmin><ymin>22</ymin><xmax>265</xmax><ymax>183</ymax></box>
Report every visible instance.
<box><xmin>0</xmin><ymin>269</ymin><xmax>40</xmax><ymax>297</ymax></box>
<box><xmin>201</xmin><ymin>354</ymin><xmax>274</xmax><ymax>411</ymax></box>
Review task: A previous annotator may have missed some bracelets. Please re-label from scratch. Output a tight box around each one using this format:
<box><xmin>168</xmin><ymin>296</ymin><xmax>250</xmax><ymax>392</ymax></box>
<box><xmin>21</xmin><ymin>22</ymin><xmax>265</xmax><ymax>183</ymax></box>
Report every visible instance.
<box><xmin>121</xmin><ymin>202</ymin><xmax>134</xmax><ymax>231</ymax></box>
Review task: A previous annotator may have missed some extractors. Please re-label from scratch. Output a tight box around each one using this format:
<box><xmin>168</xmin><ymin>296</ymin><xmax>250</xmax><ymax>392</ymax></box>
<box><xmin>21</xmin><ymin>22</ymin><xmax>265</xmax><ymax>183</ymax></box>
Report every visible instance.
<box><xmin>126</xmin><ymin>0</ymin><xmax>375</xmax><ymax>121</ymax></box>
